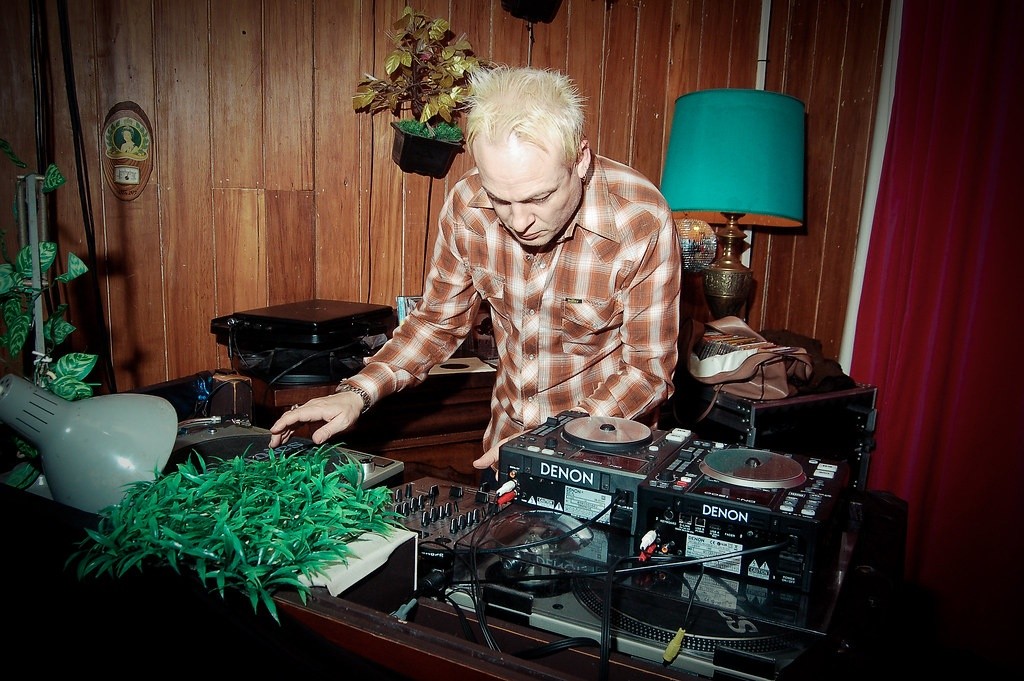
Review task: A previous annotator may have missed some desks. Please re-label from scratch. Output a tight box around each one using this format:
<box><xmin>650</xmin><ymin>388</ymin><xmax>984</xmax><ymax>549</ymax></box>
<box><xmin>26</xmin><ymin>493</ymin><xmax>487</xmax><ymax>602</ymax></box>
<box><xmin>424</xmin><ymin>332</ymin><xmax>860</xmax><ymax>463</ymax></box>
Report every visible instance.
<box><xmin>230</xmin><ymin>356</ymin><xmax>497</xmax><ymax>487</ymax></box>
<box><xmin>274</xmin><ymin>464</ymin><xmax>854</xmax><ymax>681</ymax></box>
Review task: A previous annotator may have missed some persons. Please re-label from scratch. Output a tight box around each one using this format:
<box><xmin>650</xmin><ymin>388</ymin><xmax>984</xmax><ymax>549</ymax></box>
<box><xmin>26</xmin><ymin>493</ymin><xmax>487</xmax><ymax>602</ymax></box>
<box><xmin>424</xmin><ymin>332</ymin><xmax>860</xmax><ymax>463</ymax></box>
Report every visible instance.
<box><xmin>270</xmin><ymin>68</ymin><xmax>682</xmax><ymax>510</ymax></box>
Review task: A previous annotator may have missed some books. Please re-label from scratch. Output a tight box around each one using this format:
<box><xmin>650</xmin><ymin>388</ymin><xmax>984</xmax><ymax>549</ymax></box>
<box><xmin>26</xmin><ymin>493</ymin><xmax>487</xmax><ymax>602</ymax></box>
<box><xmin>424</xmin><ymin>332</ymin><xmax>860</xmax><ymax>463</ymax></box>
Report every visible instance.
<box><xmin>398</xmin><ymin>297</ymin><xmax>423</xmax><ymax>324</ymax></box>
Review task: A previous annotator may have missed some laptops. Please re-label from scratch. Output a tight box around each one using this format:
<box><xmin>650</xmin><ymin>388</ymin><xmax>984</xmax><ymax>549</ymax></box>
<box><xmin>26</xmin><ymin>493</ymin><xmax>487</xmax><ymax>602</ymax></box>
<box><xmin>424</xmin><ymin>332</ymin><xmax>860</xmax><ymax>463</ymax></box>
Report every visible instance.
<box><xmin>233</xmin><ymin>299</ymin><xmax>393</xmax><ymax>331</ymax></box>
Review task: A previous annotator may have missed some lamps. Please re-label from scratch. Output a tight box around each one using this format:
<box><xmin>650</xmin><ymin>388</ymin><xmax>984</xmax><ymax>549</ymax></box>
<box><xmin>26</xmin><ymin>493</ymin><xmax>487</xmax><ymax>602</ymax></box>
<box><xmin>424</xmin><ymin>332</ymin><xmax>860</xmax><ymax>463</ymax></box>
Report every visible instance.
<box><xmin>659</xmin><ymin>86</ymin><xmax>808</xmax><ymax>321</ymax></box>
<box><xmin>0</xmin><ymin>174</ymin><xmax>179</xmax><ymax>517</ymax></box>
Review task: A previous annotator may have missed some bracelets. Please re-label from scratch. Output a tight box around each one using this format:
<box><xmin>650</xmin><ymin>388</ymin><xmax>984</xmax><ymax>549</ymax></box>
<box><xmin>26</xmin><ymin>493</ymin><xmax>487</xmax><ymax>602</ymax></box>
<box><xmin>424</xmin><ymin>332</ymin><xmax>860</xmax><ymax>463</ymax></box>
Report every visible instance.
<box><xmin>338</xmin><ymin>386</ymin><xmax>370</xmax><ymax>415</ymax></box>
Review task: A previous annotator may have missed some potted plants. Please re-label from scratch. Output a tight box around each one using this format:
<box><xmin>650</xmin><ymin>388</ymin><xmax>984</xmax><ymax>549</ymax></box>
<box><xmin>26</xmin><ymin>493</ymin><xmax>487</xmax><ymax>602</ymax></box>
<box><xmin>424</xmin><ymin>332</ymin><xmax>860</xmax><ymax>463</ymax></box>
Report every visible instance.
<box><xmin>353</xmin><ymin>7</ymin><xmax>494</xmax><ymax>180</ymax></box>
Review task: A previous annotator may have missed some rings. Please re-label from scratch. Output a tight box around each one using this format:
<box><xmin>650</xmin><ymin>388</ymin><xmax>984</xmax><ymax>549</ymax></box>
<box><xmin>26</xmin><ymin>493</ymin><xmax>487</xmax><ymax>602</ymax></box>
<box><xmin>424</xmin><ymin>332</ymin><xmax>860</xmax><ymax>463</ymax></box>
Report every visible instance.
<box><xmin>291</xmin><ymin>404</ymin><xmax>298</xmax><ymax>411</ymax></box>
